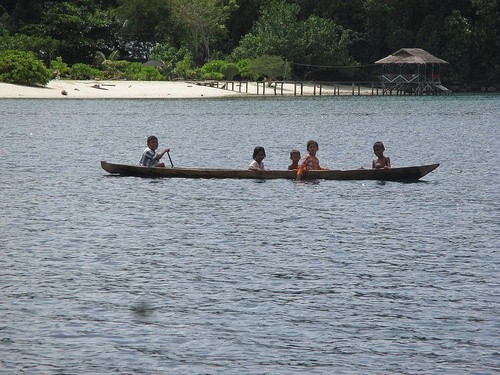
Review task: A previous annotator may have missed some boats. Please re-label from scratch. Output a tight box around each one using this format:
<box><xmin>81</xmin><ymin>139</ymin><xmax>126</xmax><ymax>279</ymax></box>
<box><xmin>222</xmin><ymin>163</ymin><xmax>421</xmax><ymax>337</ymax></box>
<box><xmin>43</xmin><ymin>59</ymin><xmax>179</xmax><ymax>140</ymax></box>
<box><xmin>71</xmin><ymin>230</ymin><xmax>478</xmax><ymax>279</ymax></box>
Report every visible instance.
<box><xmin>101</xmin><ymin>161</ymin><xmax>440</xmax><ymax>182</ymax></box>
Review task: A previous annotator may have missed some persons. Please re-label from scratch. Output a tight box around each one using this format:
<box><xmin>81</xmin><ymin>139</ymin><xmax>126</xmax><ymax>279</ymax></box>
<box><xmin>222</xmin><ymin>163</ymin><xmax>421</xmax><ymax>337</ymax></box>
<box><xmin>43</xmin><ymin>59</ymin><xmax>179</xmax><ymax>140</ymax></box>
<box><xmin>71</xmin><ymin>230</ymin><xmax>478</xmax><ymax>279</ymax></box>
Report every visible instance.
<box><xmin>372</xmin><ymin>141</ymin><xmax>390</xmax><ymax>169</ymax></box>
<box><xmin>288</xmin><ymin>150</ymin><xmax>304</xmax><ymax>171</ymax></box>
<box><xmin>139</xmin><ymin>136</ymin><xmax>170</xmax><ymax>167</ymax></box>
<box><xmin>296</xmin><ymin>141</ymin><xmax>330</xmax><ymax>181</ymax></box>
<box><xmin>249</xmin><ymin>146</ymin><xmax>267</xmax><ymax>175</ymax></box>
